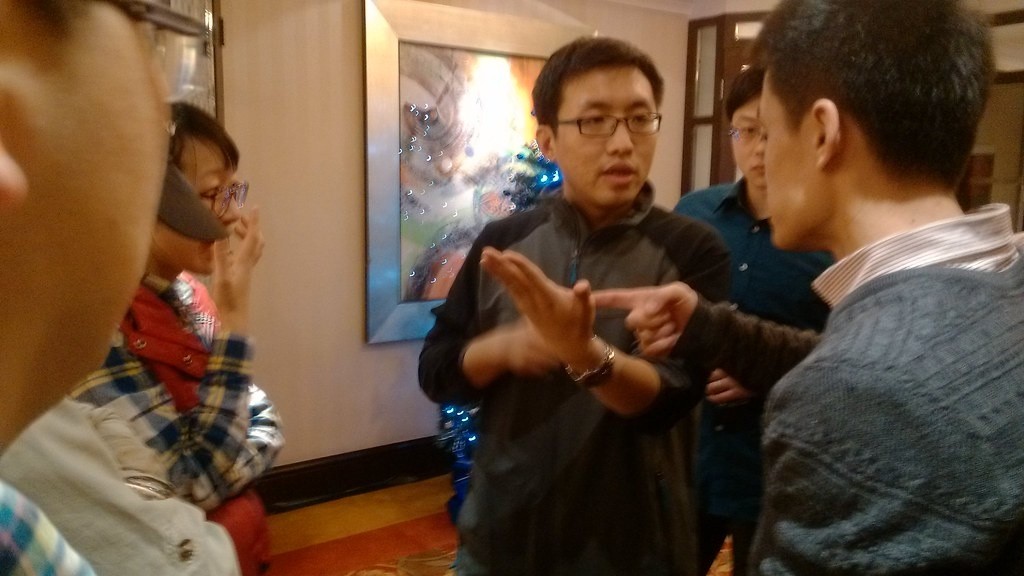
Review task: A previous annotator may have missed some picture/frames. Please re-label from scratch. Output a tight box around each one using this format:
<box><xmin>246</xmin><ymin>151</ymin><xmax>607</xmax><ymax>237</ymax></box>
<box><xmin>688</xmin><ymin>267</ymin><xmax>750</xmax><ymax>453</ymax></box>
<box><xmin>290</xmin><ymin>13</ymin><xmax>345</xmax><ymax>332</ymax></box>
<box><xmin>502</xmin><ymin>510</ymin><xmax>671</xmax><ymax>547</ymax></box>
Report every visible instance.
<box><xmin>365</xmin><ymin>0</ymin><xmax>595</xmax><ymax>345</ymax></box>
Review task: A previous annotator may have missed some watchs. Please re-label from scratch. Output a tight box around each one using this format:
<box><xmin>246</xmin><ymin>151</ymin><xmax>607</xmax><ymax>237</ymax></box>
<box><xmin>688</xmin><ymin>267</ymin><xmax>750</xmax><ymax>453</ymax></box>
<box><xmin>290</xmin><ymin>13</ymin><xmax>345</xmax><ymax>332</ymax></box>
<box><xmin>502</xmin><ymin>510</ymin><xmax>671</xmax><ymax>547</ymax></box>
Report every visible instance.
<box><xmin>565</xmin><ymin>342</ymin><xmax>615</xmax><ymax>391</ymax></box>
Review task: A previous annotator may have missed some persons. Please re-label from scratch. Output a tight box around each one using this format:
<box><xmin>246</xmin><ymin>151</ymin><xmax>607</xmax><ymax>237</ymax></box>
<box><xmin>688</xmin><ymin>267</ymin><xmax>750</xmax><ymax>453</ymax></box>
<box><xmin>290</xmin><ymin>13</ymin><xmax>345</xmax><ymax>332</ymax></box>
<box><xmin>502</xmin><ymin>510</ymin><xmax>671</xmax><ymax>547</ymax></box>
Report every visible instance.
<box><xmin>582</xmin><ymin>0</ymin><xmax>1023</xmax><ymax>576</ymax></box>
<box><xmin>0</xmin><ymin>0</ymin><xmax>210</xmax><ymax>576</ymax></box>
<box><xmin>0</xmin><ymin>397</ymin><xmax>249</xmax><ymax>575</ymax></box>
<box><xmin>414</xmin><ymin>32</ymin><xmax>738</xmax><ymax>576</ymax></box>
<box><xmin>65</xmin><ymin>98</ymin><xmax>282</xmax><ymax>575</ymax></box>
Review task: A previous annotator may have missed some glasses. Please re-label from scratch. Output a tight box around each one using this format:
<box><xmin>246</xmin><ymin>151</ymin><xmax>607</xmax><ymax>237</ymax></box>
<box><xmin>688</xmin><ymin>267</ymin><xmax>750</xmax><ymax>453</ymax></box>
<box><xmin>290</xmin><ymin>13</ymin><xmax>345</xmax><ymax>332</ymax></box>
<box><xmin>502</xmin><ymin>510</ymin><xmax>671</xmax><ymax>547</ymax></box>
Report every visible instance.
<box><xmin>554</xmin><ymin>112</ymin><xmax>662</xmax><ymax>137</ymax></box>
<box><xmin>200</xmin><ymin>179</ymin><xmax>248</xmax><ymax>216</ymax></box>
<box><xmin>110</xmin><ymin>0</ymin><xmax>214</xmax><ymax>100</ymax></box>
<box><xmin>729</xmin><ymin>124</ymin><xmax>769</xmax><ymax>143</ymax></box>
